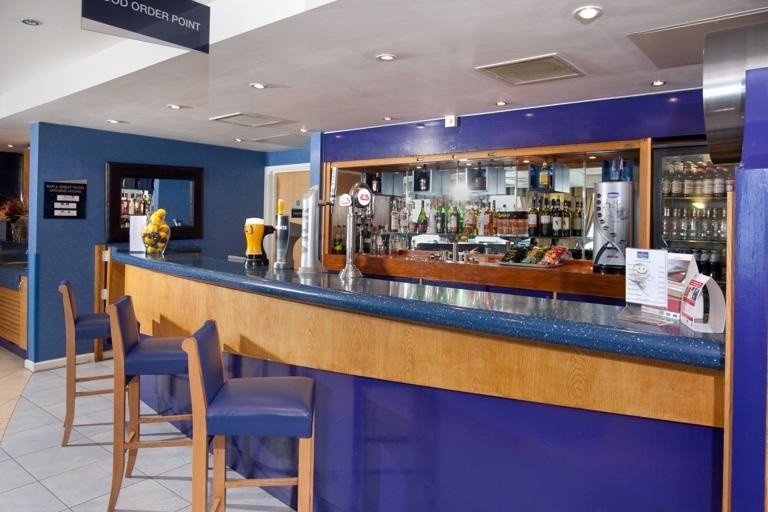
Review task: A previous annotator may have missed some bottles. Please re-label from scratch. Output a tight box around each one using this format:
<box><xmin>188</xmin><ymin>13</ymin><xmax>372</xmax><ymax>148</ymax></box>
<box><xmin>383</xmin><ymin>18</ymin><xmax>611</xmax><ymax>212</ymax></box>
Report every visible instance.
<box><xmin>539</xmin><ymin>158</ymin><xmax>552</xmax><ymax>191</ymax></box>
<box><xmin>371</xmin><ymin>170</ymin><xmax>381</xmax><ymax>192</ymax></box>
<box><xmin>389</xmin><ymin>199</ymin><xmax>399</xmax><ymax>232</ymax></box>
<box><xmin>416</xmin><ymin>196</ymin><xmax>497</xmax><ymax>237</ymax></box>
<box><xmin>120</xmin><ymin>193</ymin><xmax>153</xmax><ymax>225</ymax></box>
<box><xmin>473</xmin><ymin>160</ymin><xmax>484</xmax><ymax>189</ymax></box>
<box><xmin>663</xmin><ymin>157</ymin><xmax>733</xmax><ymax>281</ymax></box>
<box><xmin>140</xmin><ymin>209</ymin><xmax>171</xmax><ymax>258</ymax></box>
<box><xmin>334</xmin><ymin>223</ymin><xmax>388</xmax><ymax>255</ymax></box>
<box><xmin>527</xmin><ymin>197</ymin><xmax>585</xmax><ymax>238</ymax></box>
<box><xmin>418</xmin><ymin>163</ymin><xmax>428</xmax><ymax>190</ymax></box>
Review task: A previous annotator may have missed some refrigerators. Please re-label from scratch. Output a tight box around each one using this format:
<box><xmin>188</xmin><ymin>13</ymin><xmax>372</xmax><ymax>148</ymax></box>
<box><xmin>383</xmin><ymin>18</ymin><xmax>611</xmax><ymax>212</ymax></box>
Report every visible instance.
<box><xmin>651</xmin><ymin>136</ymin><xmax>738</xmax><ymax>298</ymax></box>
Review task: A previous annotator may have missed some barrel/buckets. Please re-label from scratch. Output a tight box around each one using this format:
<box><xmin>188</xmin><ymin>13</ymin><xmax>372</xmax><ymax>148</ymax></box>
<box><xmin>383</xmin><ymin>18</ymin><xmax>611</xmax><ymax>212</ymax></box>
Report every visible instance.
<box><xmin>494</xmin><ymin>210</ymin><xmax>533</xmax><ymax>238</ymax></box>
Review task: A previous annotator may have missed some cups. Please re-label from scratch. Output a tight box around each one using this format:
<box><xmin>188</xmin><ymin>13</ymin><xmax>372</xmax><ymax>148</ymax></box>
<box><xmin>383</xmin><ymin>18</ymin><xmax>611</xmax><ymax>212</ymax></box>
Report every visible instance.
<box><xmin>242</xmin><ymin>216</ymin><xmax>264</xmax><ymax>255</ymax></box>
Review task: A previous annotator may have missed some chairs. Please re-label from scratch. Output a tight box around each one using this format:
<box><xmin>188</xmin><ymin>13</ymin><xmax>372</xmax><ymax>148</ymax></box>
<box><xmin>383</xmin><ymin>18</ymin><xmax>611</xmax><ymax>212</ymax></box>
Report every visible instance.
<box><xmin>178</xmin><ymin>315</ymin><xmax>318</xmax><ymax>511</ymax></box>
<box><xmin>56</xmin><ymin>279</ymin><xmax>140</xmax><ymax>447</ymax></box>
<box><xmin>103</xmin><ymin>294</ymin><xmax>204</xmax><ymax>511</ymax></box>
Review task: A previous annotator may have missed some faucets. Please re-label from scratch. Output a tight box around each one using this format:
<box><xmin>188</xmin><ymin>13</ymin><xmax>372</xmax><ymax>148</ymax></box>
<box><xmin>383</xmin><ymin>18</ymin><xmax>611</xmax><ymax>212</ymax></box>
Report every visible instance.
<box><xmin>320</xmin><ymin>161</ymin><xmax>336</xmax><ymax>214</ymax></box>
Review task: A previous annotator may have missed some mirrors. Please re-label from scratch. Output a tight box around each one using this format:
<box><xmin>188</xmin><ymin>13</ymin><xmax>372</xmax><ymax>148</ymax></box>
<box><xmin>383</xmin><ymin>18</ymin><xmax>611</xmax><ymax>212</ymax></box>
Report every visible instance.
<box><xmin>105</xmin><ymin>162</ymin><xmax>203</xmax><ymax>242</ymax></box>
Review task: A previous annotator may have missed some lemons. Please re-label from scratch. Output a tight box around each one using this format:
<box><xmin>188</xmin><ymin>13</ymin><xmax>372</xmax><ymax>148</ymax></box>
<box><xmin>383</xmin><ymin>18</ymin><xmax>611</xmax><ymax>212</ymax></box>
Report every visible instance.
<box><xmin>144</xmin><ymin>208</ymin><xmax>170</xmax><ymax>253</ymax></box>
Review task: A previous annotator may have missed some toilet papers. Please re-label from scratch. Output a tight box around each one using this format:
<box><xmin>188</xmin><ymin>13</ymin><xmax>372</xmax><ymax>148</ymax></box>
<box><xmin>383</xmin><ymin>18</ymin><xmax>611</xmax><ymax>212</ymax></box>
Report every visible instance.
<box><xmin>128</xmin><ymin>214</ymin><xmax>148</xmax><ymax>254</ymax></box>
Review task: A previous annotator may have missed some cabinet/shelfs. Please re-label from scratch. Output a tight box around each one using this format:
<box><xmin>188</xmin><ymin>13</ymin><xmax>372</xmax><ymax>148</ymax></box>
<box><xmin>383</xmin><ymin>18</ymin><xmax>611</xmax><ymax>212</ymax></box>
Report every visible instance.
<box><xmin>650</xmin><ymin>147</ymin><xmax>739</xmax><ymax>285</ymax></box>
